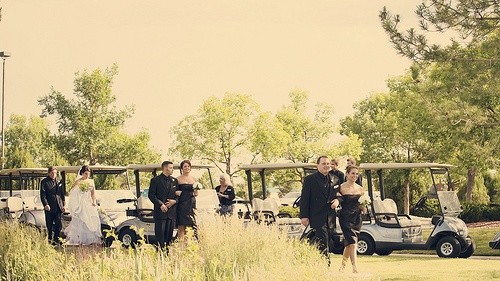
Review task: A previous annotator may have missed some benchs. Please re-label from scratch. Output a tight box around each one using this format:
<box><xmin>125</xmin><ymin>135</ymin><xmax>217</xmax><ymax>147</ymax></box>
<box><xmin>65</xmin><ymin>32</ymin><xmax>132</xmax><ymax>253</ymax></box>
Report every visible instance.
<box><xmin>62</xmin><ymin>196</ymin><xmax>72</xmax><ymax>220</ymax></box>
<box><xmin>93</xmin><ymin>189</ymin><xmax>136</xmax><ymax>213</ymax></box>
<box><xmin>0</xmin><ymin>190</ymin><xmax>21</xmax><ymax>210</ymax></box>
<box><xmin>21</xmin><ymin>190</ymin><xmax>45</xmax><ymax>210</ymax></box>
<box><xmin>195</xmin><ymin>189</ymin><xmax>220</xmax><ymax>211</ymax></box>
<box><xmin>372</xmin><ymin>198</ymin><xmax>421</xmax><ymax>227</ymax></box>
<box><xmin>251</xmin><ymin>192</ymin><xmax>303</xmax><ymax>225</ymax></box>
<box><xmin>136</xmin><ymin>195</ymin><xmax>156</xmax><ymax>216</ymax></box>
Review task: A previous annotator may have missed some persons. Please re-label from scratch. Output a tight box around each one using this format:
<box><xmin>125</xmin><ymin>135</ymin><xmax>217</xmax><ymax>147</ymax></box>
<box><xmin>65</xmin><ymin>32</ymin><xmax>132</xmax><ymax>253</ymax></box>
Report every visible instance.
<box><xmin>215</xmin><ymin>173</ymin><xmax>235</xmax><ymax>219</ymax></box>
<box><xmin>299</xmin><ymin>155</ymin><xmax>346</xmax><ymax>269</ymax></box>
<box><xmin>63</xmin><ymin>164</ymin><xmax>103</xmax><ymax>247</ymax></box>
<box><xmin>174</xmin><ymin>160</ymin><xmax>199</xmax><ymax>249</ymax></box>
<box><xmin>336</xmin><ymin>164</ymin><xmax>367</xmax><ymax>274</ymax></box>
<box><xmin>345</xmin><ymin>157</ymin><xmax>363</xmax><ymax>187</ymax></box>
<box><xmin>148</xmin><ymin>161</ymin><xmax>182</xmax><ymax>258</ymax></box>
<box><xmin>329</xmin><ymin>160</ymin><xmax>345</xmax><ymax>184</ymax></box>
<box><xmin>40</xmin><ymin>166</ymin><xmax>66</xmax><ymax>246</ymax></box>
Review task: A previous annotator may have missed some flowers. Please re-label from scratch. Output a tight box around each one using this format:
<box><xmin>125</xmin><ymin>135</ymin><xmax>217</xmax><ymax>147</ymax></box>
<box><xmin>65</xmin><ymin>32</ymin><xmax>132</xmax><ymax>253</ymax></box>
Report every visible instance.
<box><xmin>79</xmin><ymin>181</ymin><xmax>91</xmax><ymax>193</ymax></box>
<box><xmin>192</xmin><ymin>182</ymin><xmax>203</xmax><ymax>194</ymax></box>
<box><xmin>358</xmin><ymin>194</ymin><xmax>371</xmax><ymax>209</ymax></box>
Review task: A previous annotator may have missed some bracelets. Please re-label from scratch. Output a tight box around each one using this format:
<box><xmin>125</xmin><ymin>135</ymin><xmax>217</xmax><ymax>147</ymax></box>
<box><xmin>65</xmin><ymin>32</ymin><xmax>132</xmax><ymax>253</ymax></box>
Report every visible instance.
<box><xmin>175</xmin><ymin>200</ymin><xmax>177</xmax><ymax>205</ymax></box>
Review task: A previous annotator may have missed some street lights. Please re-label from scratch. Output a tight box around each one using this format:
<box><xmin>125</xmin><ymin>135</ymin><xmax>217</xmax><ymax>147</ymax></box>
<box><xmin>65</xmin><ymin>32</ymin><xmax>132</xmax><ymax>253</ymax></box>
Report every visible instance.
<box><xmin>0</xmin><ymin>51</ymin><xmax>12</xmax><ymax>167</ymax></box>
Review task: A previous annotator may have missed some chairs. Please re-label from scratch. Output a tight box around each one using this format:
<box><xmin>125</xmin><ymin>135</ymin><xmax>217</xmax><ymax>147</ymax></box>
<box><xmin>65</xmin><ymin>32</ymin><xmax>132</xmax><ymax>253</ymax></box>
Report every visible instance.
<box><xmin>6</xmin><ymin>197</ymin><xmax>24</xmax><ymax>219</ymax></box>
